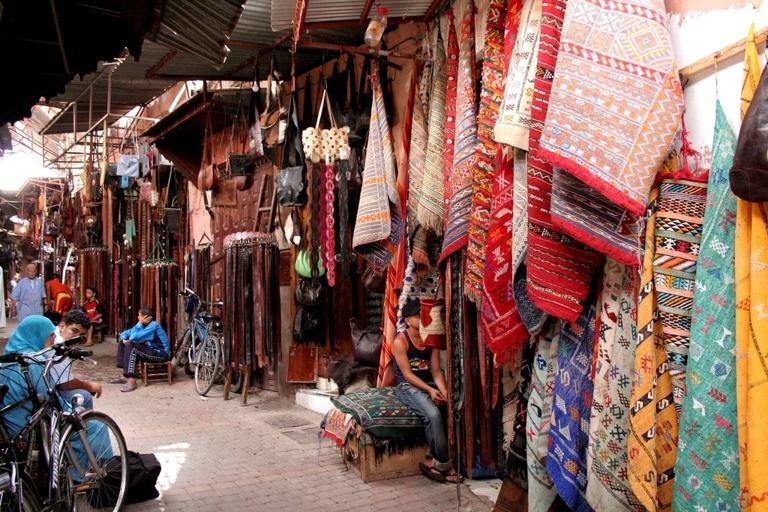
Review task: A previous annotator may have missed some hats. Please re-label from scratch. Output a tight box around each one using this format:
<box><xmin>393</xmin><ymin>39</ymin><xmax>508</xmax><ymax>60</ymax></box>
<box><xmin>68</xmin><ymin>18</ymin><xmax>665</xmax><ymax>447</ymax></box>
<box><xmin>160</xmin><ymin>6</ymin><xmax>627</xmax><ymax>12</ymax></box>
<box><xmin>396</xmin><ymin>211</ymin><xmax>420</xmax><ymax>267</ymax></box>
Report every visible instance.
<box><xmin>401</xmin><ymin>300</ymin><xmax>422</xmax><ymax>318</ymax></box>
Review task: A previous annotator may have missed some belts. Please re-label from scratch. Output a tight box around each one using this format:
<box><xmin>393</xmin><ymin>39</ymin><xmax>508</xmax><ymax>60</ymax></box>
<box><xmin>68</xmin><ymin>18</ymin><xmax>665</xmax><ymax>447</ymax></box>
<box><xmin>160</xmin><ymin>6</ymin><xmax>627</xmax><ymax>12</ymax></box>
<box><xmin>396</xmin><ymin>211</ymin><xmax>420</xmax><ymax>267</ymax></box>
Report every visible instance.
<box><xmin>222</xmin><ymin>231</ymin><xmax>284</xmax><ymax>372</ymax></box>
<box><xmin>78</xmin><ymin>248</ymin><xmax>108</xmax><ymax>310</ymax></box>
<box><xmin>139</xmin><ymin>260</ymin><xmax>180</xmax><ymax>355</ymax></box>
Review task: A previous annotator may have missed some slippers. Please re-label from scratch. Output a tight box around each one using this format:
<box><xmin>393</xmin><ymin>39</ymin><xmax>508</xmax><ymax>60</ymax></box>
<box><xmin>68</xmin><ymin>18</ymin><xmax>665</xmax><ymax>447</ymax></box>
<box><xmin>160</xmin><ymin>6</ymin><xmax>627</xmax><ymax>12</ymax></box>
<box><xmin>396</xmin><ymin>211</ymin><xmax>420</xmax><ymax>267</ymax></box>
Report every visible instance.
<box><xmin>418</xmin><ymin>462</ymin><xmax>445</xmax><ymax>484</ymax></box>
<box><xmin>433</xmin><ymin>464</ymin><xmax>464</xmax><ymax>484</ymax></box>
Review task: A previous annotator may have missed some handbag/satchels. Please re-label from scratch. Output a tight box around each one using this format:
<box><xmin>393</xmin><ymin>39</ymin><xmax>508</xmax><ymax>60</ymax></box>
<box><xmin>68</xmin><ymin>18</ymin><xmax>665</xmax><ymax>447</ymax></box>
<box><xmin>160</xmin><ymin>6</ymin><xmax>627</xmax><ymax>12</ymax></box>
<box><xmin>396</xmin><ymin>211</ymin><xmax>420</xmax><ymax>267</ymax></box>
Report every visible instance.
<box><xmin>292</xmin><ymin>306</ymin><xmax>328</xmax><ymax>347</ymax></box>
<box><xmin>86</xmin><ymin>450</ymin><xmax>161</xmax><ymax>509</ymax></box>
<box><xmin>286</xmin><ymin>344</ymin><xmax>320</xmax><ymax>385</ymax></box>
<box><xmin>348</xmin><ymin>317</ymin><xmax>385</xmax><ymax>368</ymax></box>
<box><xmin>275</xmin><ymin>93</ymin><xmax>309</xmax><ymax>209</ymax></box>
<box><xmin>292</xmin><ymin>223</ymin><xmax>327</xmax><ymax>279</ymax></box>
<box><xmin>258</xmin><ymin>74</ymin><xmax>290</xmax><ymax>150</ymax></box>
<box><xmin>301</xmin><ymin>49</ymin><xmax>380</xmax><ymax>164</ymax></box>
<box><xmin>196</xmin><ymin>116</ymin><xmax>221</xmax><ymax>193</ymax></box>
<box><xmin>294</xmin><ymin>276</ymin><xmax>331</xmax><ymax>308</ymax></box>
<box><xmin>226</xmin><ymin>98</ymin><xmax>259</xmax><ymax>178</ymax></box>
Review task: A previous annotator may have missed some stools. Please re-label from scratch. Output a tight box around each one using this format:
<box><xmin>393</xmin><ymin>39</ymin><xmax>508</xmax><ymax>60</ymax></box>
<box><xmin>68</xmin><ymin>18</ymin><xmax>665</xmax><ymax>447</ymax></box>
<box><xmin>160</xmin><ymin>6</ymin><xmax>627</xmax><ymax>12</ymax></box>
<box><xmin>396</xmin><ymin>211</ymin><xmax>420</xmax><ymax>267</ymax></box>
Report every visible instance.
<box><xmin>141</xmin><ymin>361</ymin><xmax>172</xmax><ymax>386</ymax></box>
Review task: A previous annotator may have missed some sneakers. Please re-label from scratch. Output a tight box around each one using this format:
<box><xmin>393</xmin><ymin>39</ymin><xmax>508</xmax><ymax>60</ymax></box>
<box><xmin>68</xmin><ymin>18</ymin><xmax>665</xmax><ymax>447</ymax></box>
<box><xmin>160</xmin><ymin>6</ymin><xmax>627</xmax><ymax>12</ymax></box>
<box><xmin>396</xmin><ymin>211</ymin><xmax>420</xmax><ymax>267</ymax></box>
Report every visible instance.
<box><xmin>120</xmin><ymin>383</ymin><xmax>138</xmax><ymax>392</ymax></box>
<box><xmin>110</xmin><ymin>375</ymin><xmax>128</xmax><ymax>384</ymax></box>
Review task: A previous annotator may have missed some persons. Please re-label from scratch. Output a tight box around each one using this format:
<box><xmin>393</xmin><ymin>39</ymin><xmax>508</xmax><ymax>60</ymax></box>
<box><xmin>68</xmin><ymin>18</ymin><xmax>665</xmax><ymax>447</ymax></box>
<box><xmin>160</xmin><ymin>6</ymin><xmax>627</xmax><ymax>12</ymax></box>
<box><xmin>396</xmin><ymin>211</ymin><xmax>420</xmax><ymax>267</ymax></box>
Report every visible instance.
<box><xmin>46</xmin><ymin>273</ymin><xmax>73</xmax><ymax>320</ymax></box>
<box><xmin>11</xmin><ymin>264</ymin><xmax>47</xmax><ymax>322</ymax></box>
<box><xmin>109</xmin><ymin>309</ymin><xmax>170</xmax><ymax>392</ymax></box>
<box><xmin>391</xmin><ymin>301</ymin><xmax>465</xmax><ymax>484</ymax></box>
<box><xmin>50</xmin><ymin>309</ymin><xmax>102</xmax><ymax>411</ymax></box>
<box><xmin>0</xmin><ymin>315</ymin><xmax>114</xmax><ymax>483</ymax></box>
<box><xmin>7</xmin><ymin>277</ymin><xmax>17</xmax><ymax>318</ymax></box>
<box><xmin>79</xmin><ymin>288</ymin><xmax>105</xmax><ymax>347</ymax></box>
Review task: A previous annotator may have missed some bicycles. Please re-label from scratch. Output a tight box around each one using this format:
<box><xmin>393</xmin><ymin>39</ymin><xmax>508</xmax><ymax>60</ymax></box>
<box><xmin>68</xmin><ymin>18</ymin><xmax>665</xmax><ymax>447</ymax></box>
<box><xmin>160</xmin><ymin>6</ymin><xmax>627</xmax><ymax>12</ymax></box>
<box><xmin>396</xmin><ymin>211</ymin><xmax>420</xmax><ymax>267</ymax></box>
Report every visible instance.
<box><xmin>0</xmin><ymin>336</ymin><xmax>128</xmax><ymax>510</ymax></box>
<box><xmin>170</xmin><ymin>287</ymin><xmax>224</xmax><ymax>395</ymax></box>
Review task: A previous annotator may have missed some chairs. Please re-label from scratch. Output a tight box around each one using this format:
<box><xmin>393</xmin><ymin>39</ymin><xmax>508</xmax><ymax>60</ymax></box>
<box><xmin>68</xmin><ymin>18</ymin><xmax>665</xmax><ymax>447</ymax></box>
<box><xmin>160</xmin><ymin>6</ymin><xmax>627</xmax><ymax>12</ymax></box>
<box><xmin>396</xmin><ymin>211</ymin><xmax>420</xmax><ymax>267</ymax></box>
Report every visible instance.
<box><xmin>92</xmin><ymin>330</ymin><xmax>103</xmax><ymax>342</ymax></box>
